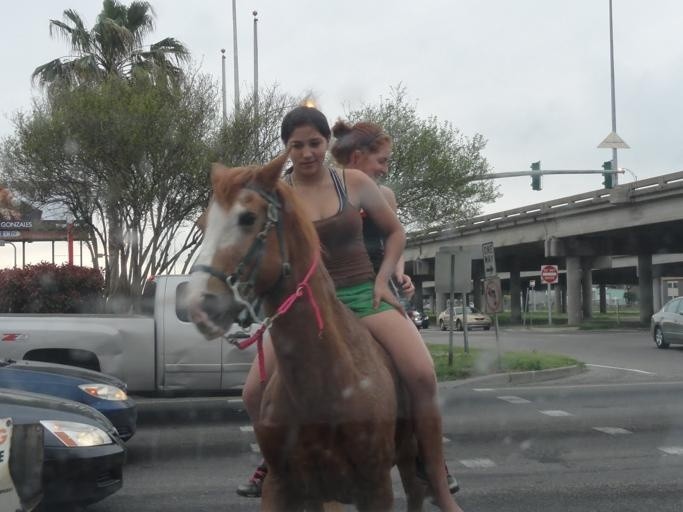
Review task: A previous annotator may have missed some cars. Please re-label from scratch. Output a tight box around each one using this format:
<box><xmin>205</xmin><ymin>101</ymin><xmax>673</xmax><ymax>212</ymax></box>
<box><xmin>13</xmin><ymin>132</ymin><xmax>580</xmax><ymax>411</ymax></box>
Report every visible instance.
<box><xmin>438</xmin><ymin>306</ymin><xmax>492</xmax><ymax>332</ymax></box>
<box><xmin>0</xmin><ymin>355</ymin><xmax>139</xmax><ymax>512</ymax></box>
<box><xmin>651</xmin><ymin>297</ymin><xmax>683</xmax><ymax>349</ymax></box>
<box><xmin>406</xmin><ymin>309</ymin><xmax>429</xmax><ymax>327</ymax></box>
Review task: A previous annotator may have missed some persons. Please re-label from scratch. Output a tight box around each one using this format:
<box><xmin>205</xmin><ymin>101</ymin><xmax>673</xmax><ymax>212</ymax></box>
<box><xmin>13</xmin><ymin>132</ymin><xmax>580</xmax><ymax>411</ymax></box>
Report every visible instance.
<box><xmin>329</xmin><ymin>117</ymin><xmax>460</xmax><ymax>493</ymax></box>
<box><xmin>234</xmin><ymin>105</ymin><xmax>464</xmax><ymax>511</ymax></box>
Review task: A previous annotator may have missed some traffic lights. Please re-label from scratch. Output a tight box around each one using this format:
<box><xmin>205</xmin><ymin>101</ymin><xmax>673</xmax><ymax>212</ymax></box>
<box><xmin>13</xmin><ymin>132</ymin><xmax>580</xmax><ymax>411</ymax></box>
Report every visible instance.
<box><xmin>601</xmin><ymin>161</ymin><xmax>613</xmax><ymax>189</ymax></box>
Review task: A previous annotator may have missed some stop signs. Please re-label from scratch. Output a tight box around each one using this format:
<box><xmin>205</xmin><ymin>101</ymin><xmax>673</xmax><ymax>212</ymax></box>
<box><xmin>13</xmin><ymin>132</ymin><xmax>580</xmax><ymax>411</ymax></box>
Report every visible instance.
<box><xmin>540</xmin><ymin>264</ymin><xmax>559</xmax><ymax>285</ymax></box>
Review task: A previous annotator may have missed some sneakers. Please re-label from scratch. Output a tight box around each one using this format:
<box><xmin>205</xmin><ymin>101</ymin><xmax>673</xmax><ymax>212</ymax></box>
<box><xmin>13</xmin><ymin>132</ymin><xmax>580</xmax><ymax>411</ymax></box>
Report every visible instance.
<box><xmin>446</xmin><ymin>472</ymin><xmax>459</xmax><ymax>493</ymax></box>
<box><xmin>236</xmin><ymin>464</ymin><xmax>268</xmax><ymax>498</ymax></box>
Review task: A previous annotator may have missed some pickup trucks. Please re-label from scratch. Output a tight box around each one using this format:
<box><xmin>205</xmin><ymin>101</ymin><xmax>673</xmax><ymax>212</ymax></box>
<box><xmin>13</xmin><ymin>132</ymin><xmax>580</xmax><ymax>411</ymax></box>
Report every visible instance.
<box><xmin>0</xmin><ymin>273</ymin><xmax>266</xmax><ymax>394</ymax></box>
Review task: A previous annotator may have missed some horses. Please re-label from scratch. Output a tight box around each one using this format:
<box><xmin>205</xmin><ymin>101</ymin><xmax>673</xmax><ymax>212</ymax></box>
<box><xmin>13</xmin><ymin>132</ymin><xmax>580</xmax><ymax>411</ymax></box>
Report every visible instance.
<box><xmin>173</xmin><ymin>141</ymin><xmax>429</xmax><ymax>512</ymax></box>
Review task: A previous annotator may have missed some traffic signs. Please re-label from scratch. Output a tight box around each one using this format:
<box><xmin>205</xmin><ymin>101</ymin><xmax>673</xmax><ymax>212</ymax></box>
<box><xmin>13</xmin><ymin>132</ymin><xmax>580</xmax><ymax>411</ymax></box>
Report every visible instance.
<box><xmin>481</xmin><ymin>241</ymin><xmax>496</xmax><ymax>277</ymax></box>
